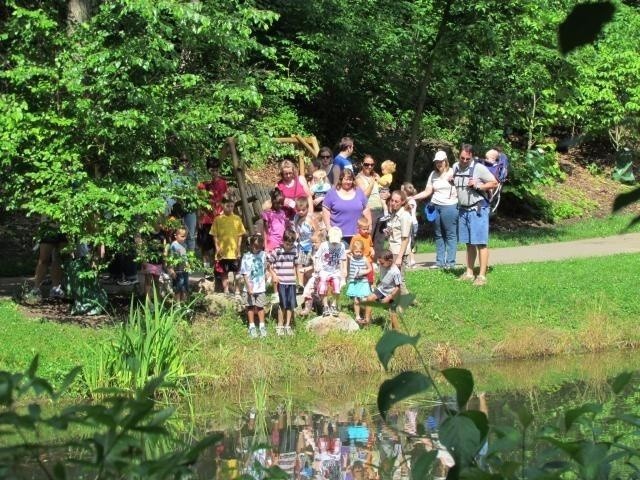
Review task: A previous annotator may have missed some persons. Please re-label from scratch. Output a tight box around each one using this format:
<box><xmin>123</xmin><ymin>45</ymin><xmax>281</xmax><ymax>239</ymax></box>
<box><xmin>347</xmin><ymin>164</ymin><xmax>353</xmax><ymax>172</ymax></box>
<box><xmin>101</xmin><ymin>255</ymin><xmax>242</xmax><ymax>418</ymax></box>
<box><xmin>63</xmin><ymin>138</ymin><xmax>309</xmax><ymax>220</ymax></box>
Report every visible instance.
<box><xmin>194</xmin><ymin>394</ymin><xmax>489</xmax><ymax>480</ymax></box>
<box><xmin>409</xmin><ymin>148</ymin><xmax>459</xmax><ymax>272</ymax></box>
<box><xmin>483</xmin><ymin>148</ymin><xmax>501</xmax><ymax>203</ymax></box>
<box><xmin>449</xmin><ymin>143</ymin><xmax>500</xmax><ymax>288</ymax></box>
<box><xmin>21</xmin><ymin>160</ymin><xmax>247</xmax><ymax>318</ymax></box>
<box><xmin>237</xmin><ymin>138</ymin><xmax>418</xmax><ymax>338</ymax></box>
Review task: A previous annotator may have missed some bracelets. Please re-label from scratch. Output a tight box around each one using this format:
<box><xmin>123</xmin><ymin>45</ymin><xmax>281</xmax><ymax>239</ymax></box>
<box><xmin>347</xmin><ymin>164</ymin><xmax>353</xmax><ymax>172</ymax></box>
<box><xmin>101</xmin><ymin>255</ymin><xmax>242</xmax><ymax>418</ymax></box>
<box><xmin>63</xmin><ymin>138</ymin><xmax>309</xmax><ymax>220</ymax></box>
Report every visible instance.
<box><xmin>476</xmin><ymin>182</ymin><xmax>480</xmax><ymax>190</ymax></box>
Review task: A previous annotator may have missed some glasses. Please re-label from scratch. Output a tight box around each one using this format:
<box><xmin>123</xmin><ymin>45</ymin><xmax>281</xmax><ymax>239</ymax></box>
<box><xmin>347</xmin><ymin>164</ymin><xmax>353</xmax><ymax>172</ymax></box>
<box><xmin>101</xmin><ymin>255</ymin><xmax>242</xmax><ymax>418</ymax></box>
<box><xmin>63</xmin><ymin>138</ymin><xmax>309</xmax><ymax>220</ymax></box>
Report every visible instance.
<box><xmin>320</xmin><ymin>156</ymin><xmax>331</xmax><ymax>159</ymax></box>
<box><xmin>461</xmin><ymin>157</ymin><xmax>471</xmax><ymax>161</ymax></box>
<box><xmin>363</xmin><ymin>163</ymin><xmax>375</xmax><ymax>167</ymax></box>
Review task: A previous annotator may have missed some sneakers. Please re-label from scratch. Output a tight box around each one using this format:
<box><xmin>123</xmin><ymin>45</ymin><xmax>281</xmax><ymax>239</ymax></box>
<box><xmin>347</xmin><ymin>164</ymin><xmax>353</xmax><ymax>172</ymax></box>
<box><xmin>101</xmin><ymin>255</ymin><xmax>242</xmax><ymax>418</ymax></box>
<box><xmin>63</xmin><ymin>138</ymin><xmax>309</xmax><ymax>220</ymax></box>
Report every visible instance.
<box><xmin>445</xmin><ymin>265</ymin><xmax>454</xmax><ymax>269</ymax></box>
<box><xmin>430</xmin><ymin>264</ymin><xmax>441</xmax><ymax>269</ymax></box>
<box><xmin>286</xmin><ymin>327</ymin><xmax>294</xmax><ymax>335</ymax></box>
<box><xmin>331</xmin><ymin>306</ymin><xmax>339</xmax><ymax>317</ymax></box>
<box><xmin>260</xmin><ymin>328</ymin><xmax>266</xmax><ymax>337</ymax></box>
<box><xmin>456</xmin><ymin>273</ymin><xmax>475</xmax><ymax>281</ymax></box>
<box><xmin>323</xmin><ymin>305</ymin><xmax>330</xmax><ymax>317</ymax></box>
<box><xmin>409</xmin><ymin>263</ymin><xmax>416</xmax><ymax>268</ymax></box>
<box><xmin>472</xmin><ymin>276</ymin><xmax>488</xmax><ymax>286</ymax></box>
<box><xmin>364</xmin><ymin>318</ymin><xmax>372</xmax><ymax>326</ymax></box>
<box><xmin>249</xmin><ymin>328</ymin><xmax>258</xmax><ymax>338</ymax></box>
<box><xmin>356</xmin><ymin>318</ymin><xmax>363</xmax><ymax>326</ymax></box>
<box><xmin>276</xmin><ymin>327</ymin><xmax>284</xmax><ymax>336</ymax></box>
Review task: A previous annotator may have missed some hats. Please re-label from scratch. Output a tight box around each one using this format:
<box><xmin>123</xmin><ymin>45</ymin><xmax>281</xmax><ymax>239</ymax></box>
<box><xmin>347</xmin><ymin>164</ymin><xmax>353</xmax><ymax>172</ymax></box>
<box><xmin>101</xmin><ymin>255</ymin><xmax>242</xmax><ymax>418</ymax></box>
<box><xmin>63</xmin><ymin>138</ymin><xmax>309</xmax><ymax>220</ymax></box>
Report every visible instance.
<box><xmin>329</xmin><ymin>226</ymin><xmax>342</xmax><ymax>244</ymax></box>
<box><xmin>425</xmin><ymin>204</ymin><xmax>436</xmax><ymax>221</ymax></box>
<box><xmin>433</xmin><ymin>151</ymin><xmax>447</xmax><ymax>161</ymax></box>
